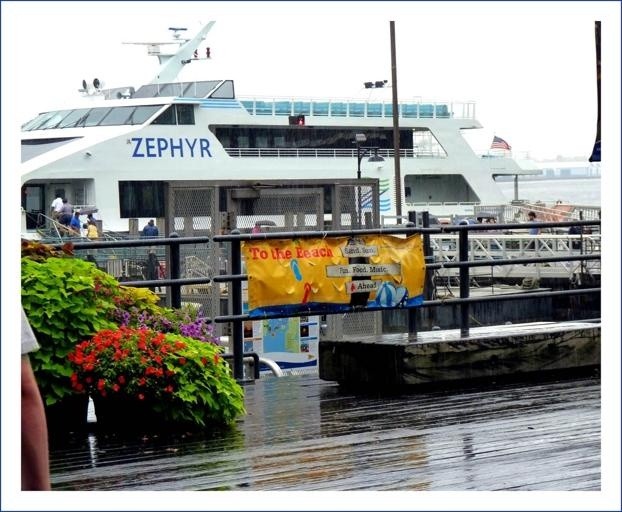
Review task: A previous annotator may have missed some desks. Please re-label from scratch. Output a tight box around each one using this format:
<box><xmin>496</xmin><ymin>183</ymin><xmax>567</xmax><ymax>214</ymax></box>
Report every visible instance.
<box><xmin>355</xmin><ymin>18</ymin><xmax>403</xmax><ymax>230</ymax></box>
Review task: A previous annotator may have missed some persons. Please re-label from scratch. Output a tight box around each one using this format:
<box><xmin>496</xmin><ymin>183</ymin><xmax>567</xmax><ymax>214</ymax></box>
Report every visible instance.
<box><xmin>528</xmin><ymin>211</ymin><xmax>541</xmax><ymax>248</ymax></box>
<box><xmin>139</xmin><ymin>219</ymin><xmax>158</xmax><ymax>238</ymax></box>
<box><xmin>567</xmin><ymin>219</ymin><xmax>581</xmax><ymax>249</ymax></box>
<box><xmin>148</xmin><ymin>253</ymin><xmax>165</xmax><ymax>292</ymax></box>
<box><xmin>22</xmin><ymin>303</ymin><xmax>51</xmax><ymax>491</ymax></box>
<box><xmin>50</xmin><ymin>193</ymin><xmax>98</xmax><ymax>239</ymax></box>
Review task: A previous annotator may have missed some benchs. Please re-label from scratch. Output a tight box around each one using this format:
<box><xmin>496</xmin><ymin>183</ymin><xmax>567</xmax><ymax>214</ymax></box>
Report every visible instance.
<box><xmin>490</xmin><ymin>136</ymin><xmax>511</xmax><ymax>150</ymax></box>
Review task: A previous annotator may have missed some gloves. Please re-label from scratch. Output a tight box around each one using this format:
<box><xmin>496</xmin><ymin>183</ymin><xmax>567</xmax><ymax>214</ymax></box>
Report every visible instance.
<box><xmin>19</xmin><ymin>21</ymin><xmax>601</xmax><ymax>360</ymax></box>
